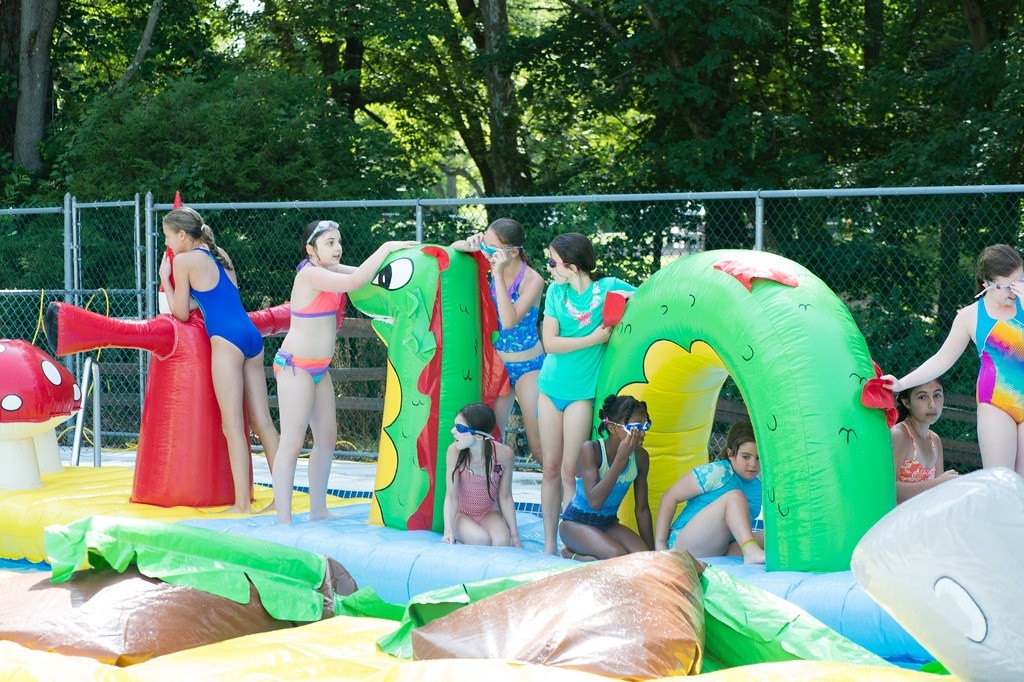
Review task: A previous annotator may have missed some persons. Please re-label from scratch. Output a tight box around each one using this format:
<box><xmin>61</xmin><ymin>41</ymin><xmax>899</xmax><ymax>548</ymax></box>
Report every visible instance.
<box><xmin>537</xmin><ymin>232</ymin><xmax>637</xmax><ymax>554</ymax></box>
<box><xmin>440</xmin><ymin>402</ymin><xmax>526</xmax><ymax>547</ymax></box>
<box><xmin>558</xmin><ymin>394</ymin><xmax>654</xmax><ymax>562</ymax></box>
<box><xmin>272</xmin><ymin>219</ymin><xmax>421</xmax><ymax>525</ymax></box>
<box><xmin>158</xmin><ymin>207</ymin><xmax>279</xmax><ymax>515</ymax></box>
<box><xmin>450</xmin><ymin>216</ymin><xmax>543</xmax><ymax>548</ymax></box>
<box><xmin>655</xmin><ymin>421</ymin><xmax>766</xmax><ymax>565</ymax></box>
<box><xmin>889</xmin><ymin>367</ymin><xmax>962</xmax><ymax>507</ymax></box>
<box><xmin>878</xmin><ymin>244</ymin><xmax>1024</xmax><ymax>479</ymax></box>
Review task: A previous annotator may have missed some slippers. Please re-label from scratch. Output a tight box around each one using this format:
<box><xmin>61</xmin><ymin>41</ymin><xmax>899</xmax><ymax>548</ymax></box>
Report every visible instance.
<box><xmin>607</xmin><ymin>421</ymin><xmax>650</xmax><ymax>435</ymax></box>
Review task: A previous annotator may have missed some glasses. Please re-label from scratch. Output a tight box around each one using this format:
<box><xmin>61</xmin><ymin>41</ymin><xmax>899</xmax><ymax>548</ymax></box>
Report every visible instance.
<box><xmin>547</xmin><ymin>257</ymin><xmax>571</xmax><ymax>269</ymax></box>
<box><xmin>974</xmin><ymin>277</ymin><xmax>1024</xmax><ymax>299</ymax></box>
<box><xmin>306</xmin><ymin>219</ymin><xmax>339</xmax><ymax>244</ymax></box>
<box><xmin>454</xmin><ymin>423</ymin><xmax>495</xmax><ymax>440</ymax></box>
<box><xmin>479</xmin><ymin>240</ymin><xmax>524</xmax><ymax>258</ymax></box>
